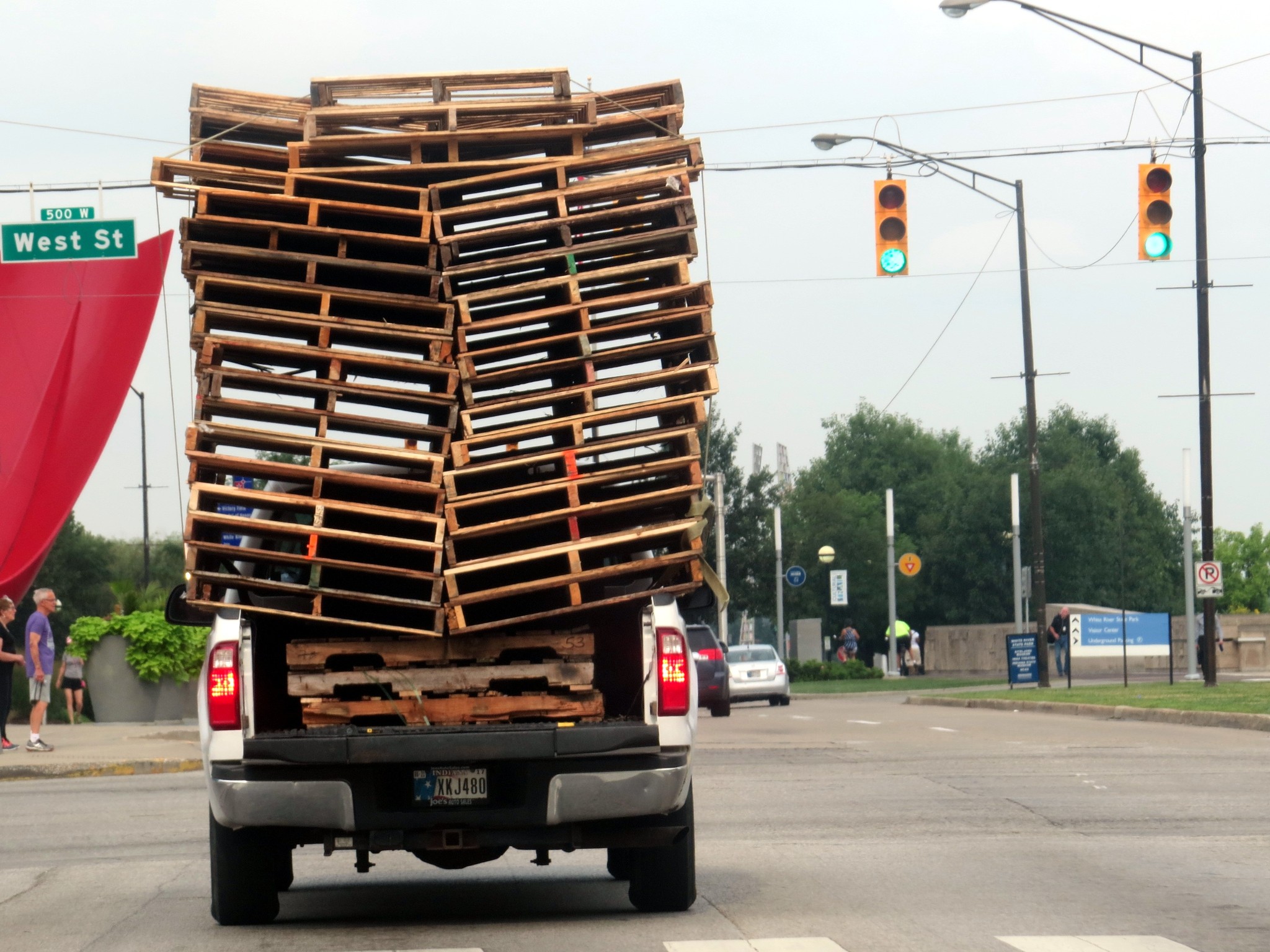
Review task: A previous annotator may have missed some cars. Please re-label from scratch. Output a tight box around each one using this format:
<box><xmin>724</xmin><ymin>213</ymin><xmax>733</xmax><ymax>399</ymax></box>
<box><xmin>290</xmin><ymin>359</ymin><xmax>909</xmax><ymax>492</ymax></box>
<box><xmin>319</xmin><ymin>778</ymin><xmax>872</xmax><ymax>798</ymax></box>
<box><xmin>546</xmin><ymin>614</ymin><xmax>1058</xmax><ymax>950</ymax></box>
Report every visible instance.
<box><xmin>724</xmin><ymin>643</ymin><xmax>791</xmax><ymax>707</ymax></box>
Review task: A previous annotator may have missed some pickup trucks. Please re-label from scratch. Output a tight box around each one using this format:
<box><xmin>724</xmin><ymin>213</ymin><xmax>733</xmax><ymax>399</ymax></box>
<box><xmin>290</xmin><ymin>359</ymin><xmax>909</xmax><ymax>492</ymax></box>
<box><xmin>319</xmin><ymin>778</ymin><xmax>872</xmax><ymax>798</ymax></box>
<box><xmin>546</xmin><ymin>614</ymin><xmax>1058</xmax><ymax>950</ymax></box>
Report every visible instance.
<box><xmin>164</xmin><ymin>458</ymin><xmax>701</xmax><ymax>925</ymax></box>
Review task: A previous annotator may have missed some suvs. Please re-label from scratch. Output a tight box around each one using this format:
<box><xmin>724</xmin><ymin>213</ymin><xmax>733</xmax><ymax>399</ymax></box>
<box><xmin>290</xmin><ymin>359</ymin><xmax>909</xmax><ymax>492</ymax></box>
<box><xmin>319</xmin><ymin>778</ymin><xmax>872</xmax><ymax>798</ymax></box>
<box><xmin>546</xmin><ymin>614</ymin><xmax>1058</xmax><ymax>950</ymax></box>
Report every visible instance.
<box><xmin>684</xmin><ymin>621</ymin><xmax>732</xmax><ymax>719</ymax></box>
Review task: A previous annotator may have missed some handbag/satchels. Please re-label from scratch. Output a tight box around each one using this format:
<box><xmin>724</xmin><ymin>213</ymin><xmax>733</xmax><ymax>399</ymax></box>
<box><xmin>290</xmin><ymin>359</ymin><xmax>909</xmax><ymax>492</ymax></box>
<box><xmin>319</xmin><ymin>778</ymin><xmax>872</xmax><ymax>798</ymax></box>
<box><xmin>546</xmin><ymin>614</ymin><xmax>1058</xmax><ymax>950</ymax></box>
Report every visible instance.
<box><xmin>1047</xmin><ymin>626</ymin><xmax>1059</xmax><ymax>643</ymax></box>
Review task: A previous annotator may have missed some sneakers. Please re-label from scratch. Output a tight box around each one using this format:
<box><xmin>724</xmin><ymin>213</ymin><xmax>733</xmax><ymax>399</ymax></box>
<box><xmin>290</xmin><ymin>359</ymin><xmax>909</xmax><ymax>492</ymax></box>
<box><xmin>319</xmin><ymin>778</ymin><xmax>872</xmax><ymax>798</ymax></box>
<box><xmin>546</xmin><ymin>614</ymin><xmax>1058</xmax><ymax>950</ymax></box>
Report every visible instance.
<box><xmin>26</xmin><ymin>739</ymin><xmax>53</xmax><ymax>751</ymax></box>
<box><xmin>2</xmin><ymin>738</ymin><xmax>20</xmax><ymax>750</ymax></box>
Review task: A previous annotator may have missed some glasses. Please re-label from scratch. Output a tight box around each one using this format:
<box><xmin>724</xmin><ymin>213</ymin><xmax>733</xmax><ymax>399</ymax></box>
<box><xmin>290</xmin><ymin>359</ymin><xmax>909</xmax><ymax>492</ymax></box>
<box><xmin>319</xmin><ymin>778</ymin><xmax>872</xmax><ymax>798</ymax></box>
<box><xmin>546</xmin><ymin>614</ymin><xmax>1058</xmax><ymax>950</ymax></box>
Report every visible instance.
<box><xmin>44</xmin><ymin>598</ymin><xmax>56</xmax><ymax>601</ymax></box>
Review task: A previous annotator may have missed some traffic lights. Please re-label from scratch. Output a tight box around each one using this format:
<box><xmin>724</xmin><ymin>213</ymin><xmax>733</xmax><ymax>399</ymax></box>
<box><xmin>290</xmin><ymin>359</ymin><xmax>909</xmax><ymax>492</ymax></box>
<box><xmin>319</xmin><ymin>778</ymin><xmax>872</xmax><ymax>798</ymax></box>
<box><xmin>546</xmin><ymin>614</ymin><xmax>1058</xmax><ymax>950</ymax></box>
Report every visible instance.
<box><xmin>1138</xmin><ymin>163</ymin><xmax>1173</xmax><ymax>263</ymax></box>
<box><xmin>873</xmin><ymin>178</ymin><xmax>909</xmax><ymax>276</ymax></box>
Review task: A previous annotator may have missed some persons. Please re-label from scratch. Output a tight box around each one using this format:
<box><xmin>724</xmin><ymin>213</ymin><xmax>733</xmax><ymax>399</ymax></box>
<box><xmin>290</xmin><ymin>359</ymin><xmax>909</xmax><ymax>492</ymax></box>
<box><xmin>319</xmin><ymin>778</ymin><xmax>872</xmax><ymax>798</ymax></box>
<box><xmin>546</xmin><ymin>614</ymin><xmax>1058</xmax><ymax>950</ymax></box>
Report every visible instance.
<box><xmin>1195</xmin><ymin>605</ymin><xmax>1224</xmax><ymax>681</ymax></box>
<box><xmin>838</xmin><ymin>617</ymin><xmax>924</xmax><ymax>676</ymax></box>
<box><xmin>1048</xmin><ymin>606</ymin><xmax>1072</xmax><ymax>679</ymax></box>
<box><xmin>0</xmin><ymin>587</ymin><xmax>86</xmax><ymax>753</ymax></box>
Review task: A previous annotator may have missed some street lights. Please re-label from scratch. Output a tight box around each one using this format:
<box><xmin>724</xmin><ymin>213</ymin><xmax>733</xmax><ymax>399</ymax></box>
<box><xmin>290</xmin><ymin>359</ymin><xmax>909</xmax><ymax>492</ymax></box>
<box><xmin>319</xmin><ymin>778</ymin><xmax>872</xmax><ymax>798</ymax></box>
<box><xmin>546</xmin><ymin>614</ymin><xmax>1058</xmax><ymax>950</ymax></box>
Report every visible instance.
<box><xmin>939</xmin><ymin>1</ymin><xmax>1219</xmax><ymax>688</ymax></box>
<box><xmin>812</xmin><ymin>133</ymin><xmax>1052</xmax><ymax>688</ymax></box>
